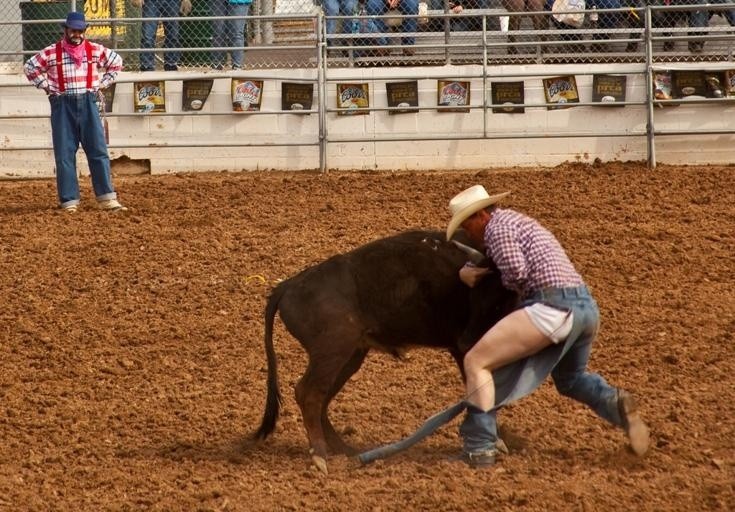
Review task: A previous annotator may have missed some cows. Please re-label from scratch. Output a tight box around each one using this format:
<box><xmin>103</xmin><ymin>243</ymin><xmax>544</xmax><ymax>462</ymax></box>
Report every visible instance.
<box><xmin>236</xmin><ymin>227</ymin><xmax>531</xmax><ymax>478</ymax></box>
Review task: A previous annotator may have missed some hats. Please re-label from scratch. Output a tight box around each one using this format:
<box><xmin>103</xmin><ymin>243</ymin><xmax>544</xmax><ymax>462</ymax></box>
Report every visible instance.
<box><xmin>61</xmin><ymin>12</ymin><xmax>90</xmax><ymax>30</ymax></box>
<box><xmin>444</xmin><ymin>184</ymin><xmax>513</xmax><ymax>242</ymax></box>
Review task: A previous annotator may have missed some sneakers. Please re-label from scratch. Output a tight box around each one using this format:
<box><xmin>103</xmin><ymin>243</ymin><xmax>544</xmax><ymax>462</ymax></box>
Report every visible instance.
<box><xmin>99</xmin><ymin>198</ymin><xmax>128</xmax><ymax>212</ymax></box>
<box><xmin>618</xmin><ymin>390</ymin><xmax>651</xmax><ymax>458</ymax></box>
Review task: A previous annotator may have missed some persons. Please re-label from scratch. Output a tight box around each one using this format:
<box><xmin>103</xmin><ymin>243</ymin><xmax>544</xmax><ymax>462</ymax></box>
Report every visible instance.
<box><xmin>22</xmin><ymin>12</ymin><xmax>126</xmax><ymax>212</ymax></box>
<box><xmin>210</xmin><ymin>0</ymin><xmax>252</xmax><ymax>69</ymax></box>
<box><xmin>131</xmin><ymin>0</ymin><xmax>193</xmax><ymax>72</ymax></box>
<box><xmin>443</xmin><ymin>183</ymin><xmax>651</xmax><ymax>471</ymax></box>
